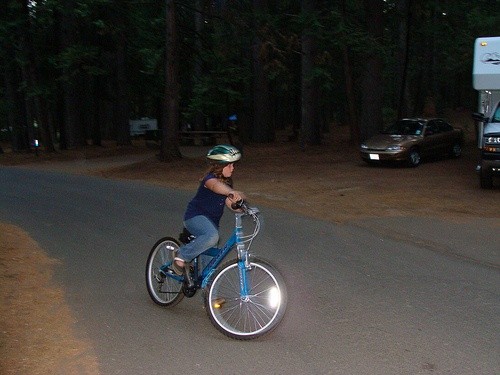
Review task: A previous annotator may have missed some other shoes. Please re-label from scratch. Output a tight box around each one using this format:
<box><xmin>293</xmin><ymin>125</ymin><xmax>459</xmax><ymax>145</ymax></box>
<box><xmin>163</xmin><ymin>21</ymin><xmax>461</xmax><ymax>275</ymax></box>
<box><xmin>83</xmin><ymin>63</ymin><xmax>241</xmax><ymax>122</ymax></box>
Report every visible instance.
<box><xmin>172</xmin><ymin>249</ymin><xmax>184</xmax><ymax>276</ymax></box>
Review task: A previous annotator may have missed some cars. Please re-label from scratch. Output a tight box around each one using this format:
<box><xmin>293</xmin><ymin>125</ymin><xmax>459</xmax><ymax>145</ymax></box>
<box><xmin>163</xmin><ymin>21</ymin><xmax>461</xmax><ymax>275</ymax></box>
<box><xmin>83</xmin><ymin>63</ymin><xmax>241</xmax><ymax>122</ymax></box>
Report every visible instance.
<box><xmin>358</xmin><ymin>117</ymin><xmax>464</xmax><ymax>167</ymax></box>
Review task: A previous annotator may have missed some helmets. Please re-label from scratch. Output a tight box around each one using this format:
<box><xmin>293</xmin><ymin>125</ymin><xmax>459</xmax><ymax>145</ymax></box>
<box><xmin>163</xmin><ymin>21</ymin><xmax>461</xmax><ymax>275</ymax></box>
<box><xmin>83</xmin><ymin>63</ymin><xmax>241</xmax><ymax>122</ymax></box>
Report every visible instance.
<box><xmin>206</xmin><ymin>144</ymin><xmax>242</xmax><ymax>164</ymax></box>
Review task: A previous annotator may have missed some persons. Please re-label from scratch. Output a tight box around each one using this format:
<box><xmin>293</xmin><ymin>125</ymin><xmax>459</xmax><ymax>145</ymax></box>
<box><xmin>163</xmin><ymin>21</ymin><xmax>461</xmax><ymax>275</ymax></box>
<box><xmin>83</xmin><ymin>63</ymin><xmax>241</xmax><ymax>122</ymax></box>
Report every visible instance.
<box><xmin>171</xmin><ymin>144</ymin><xmax>249</xmax><ymax>307</ymax></box>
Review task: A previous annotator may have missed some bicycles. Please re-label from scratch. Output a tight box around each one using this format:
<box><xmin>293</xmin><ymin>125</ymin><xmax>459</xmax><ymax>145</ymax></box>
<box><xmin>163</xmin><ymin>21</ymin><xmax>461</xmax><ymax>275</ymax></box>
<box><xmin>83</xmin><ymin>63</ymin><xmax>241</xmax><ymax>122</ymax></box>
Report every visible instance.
<box><xmin>146</xmin><ymin>194</ymin><xmax>288</xmax><ymax>340</ymax></box>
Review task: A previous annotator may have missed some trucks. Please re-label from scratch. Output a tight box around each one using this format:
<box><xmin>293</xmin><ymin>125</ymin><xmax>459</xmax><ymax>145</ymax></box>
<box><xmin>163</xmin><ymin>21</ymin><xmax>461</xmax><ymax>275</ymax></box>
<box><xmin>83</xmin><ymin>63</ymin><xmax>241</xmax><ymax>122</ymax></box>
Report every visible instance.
<box><xmin>472</xmin><ymin>37</ymin><xmax>499</xmax><ymax>189</ymax></box>
